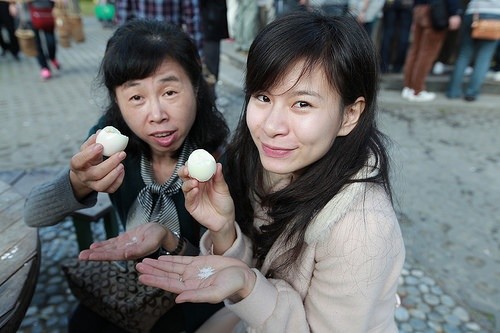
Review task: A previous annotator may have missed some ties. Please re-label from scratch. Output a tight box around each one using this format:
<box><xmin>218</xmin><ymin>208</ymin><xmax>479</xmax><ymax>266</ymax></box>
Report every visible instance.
<box><xmin>137</xmin><ymin>142</ymin><xmax>201</xmax><ymax>235</ymax></box>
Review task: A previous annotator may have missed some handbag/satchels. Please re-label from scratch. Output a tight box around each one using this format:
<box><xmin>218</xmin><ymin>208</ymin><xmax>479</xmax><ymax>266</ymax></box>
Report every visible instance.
<box><xmin>471</xmin><ymin>19</ymin><xmax>500</xmax><ymax>40</ymax></box>
<box><xmin>31</xmin><ymin>7</ymin><xmax>54</xmax><ymax>30</ymax></box>
<box><xmin>430</xmin><ymin>0</ymin><xmax>451</xmax><ymax>32</ymax></box>
<box><xmin>61</xmin><ymin>245</ymin><xmax>178</xmax><ymax>333</ymax></box>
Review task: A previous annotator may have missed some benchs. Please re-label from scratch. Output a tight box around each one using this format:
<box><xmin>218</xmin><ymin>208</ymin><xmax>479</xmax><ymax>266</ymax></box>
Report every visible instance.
<box><xmin>0</xmin><ymin>169</ymin><xmax>119</xmax><ymax>257</ymax></box>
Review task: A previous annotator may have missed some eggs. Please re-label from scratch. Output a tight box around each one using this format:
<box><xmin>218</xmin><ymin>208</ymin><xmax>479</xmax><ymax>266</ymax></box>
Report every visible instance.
<box><xmin>96</xmin><ymin>125</ymin><xmax>129</xmax><ymax>157</ymax></box>
<box><xmin>187</xmin><ymin>149</ymin><xmax>217</xmax><ymax>183</ymax></box>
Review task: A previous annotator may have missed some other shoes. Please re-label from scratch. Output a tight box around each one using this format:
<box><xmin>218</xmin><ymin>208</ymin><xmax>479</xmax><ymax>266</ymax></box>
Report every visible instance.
<box><xmin>402</xmin><ymin>87</ymin><xmax>436</xmax><ymax>103</ymax></box>
<box><xmin>51</xmin><ymin>60</ymin><xmax>59</xmax><ymax>69</ymax></box>
<box><xmin>43</xmin><ymin>68</ymin><xmax>50</xmax><ymax>78</ymax></box>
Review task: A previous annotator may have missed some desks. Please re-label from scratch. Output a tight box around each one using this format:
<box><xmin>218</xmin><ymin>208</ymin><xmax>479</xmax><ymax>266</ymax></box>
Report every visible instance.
<box><xmin>0</xmin><ymin>179</ymin><xmax>41</xmax><ymax>333</ymax></box>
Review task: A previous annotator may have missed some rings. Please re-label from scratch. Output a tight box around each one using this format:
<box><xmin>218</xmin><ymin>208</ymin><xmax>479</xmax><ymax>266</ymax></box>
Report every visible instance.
<box><xmin>179</xmin><ymin>275</ymin><xmax>183</xmax><ymax>281</ymax></box>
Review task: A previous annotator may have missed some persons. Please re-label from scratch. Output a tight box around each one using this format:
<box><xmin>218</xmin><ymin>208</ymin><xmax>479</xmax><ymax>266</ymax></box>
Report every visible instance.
<box><xmin>136</xmin><ymin>10</ymin><xmax>405</xmax><ymax>333</ymax></box>
<box><xmin>0</xmin><ymin>0</ymin><xmax>496</xmax><ymax>104</ymax></box>
<box><xmin>20</xmin><ymin>18</ymin><xmax>242</xmax><ymax>333</ymax></box>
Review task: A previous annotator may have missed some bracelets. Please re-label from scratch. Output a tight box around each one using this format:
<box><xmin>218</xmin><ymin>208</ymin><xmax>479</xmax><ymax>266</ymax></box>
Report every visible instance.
<box><xmin>161</xmin><ymin>230</ymin><xmax>184</xmax><ymax>255</ymax></box>
